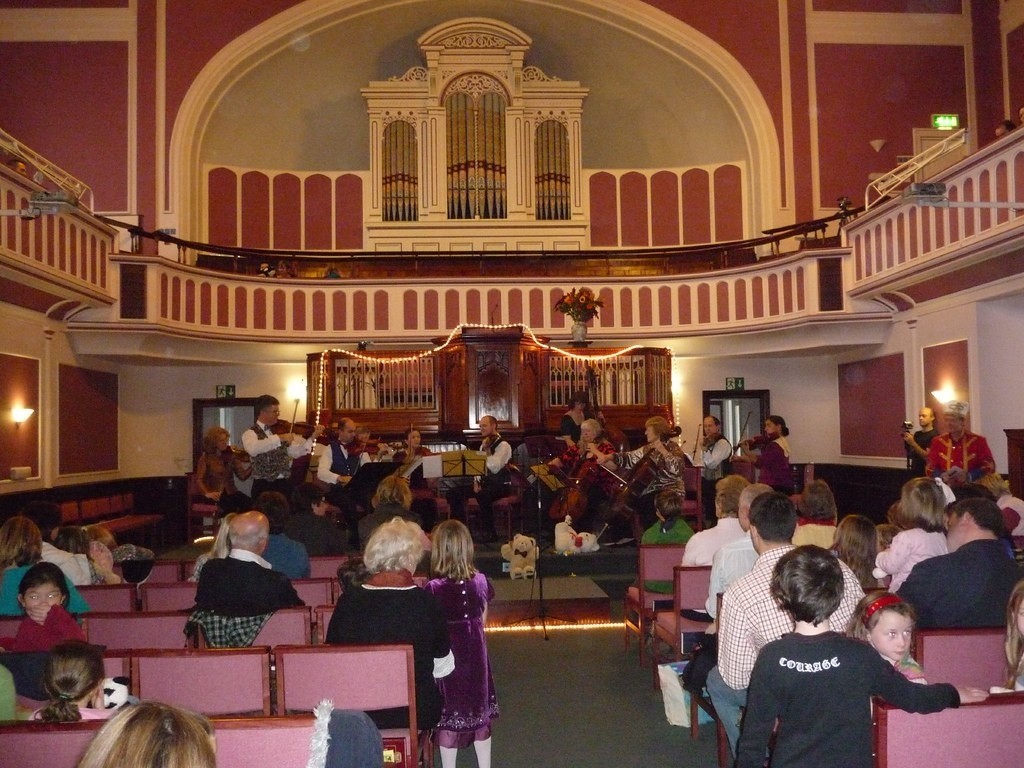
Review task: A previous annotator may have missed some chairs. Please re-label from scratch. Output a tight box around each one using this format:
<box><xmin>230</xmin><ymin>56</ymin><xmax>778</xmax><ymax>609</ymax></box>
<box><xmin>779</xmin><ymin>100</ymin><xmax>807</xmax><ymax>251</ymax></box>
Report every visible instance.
<box><xmin>467</xmin><ymin>475</ymin><xmax>525</xmax><ymax>541</ymax></box>
<box><xmin>674</xmin><ymin>467</ymin><xmax>704</xmax><ymax>532</ymax></box>
<box><xmin>0</xmin><ymin>555</ymin><xmax>418</xmax><ymax>767</ymax></box>
<box><xmin>787</xmin><ymin>462</ymin><xmax>814</xmax><ymax>510</ymax></box>
<box><xmin>57</xmin><ymin>493</ymin><xmax>165</xmax><ymax>538</ymax></box>
<box><xmin>622</xmin><ymin>542</ymin><xmax>740</xmax><ymax>768</ymax></box>
<box><xmin>870</xmin><ymin>627</ymin><xmax>1024</xmax><ymax>768</ymax></box>
<box><xmin>185</xmin><ymin>471</ymin><xmax>219</xmax><ymax>548</ymax></box>
<box><xmin>727</xmin><ymin>459</ymin><xmax>756</xmax><ymax>485</ymax></box>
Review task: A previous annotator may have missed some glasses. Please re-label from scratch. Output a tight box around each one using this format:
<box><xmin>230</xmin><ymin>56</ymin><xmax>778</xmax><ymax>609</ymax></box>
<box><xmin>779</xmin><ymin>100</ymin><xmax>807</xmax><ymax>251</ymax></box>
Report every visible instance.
<box><xmin>264</xmin><ymin>409</ymin><xmax>280</xmax><ymax>416</ymax></box>
<box><xmin>29</xmin><ymin>592</ymin><xmax>62</xmax><ymax>600</ymax></box>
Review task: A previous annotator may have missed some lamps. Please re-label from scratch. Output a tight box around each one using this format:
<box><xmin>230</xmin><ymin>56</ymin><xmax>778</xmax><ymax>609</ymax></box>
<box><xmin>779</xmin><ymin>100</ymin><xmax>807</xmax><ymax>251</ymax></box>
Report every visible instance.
<box><xmin>871</xmin><ymin>139</ymin><xmax>886</xmax><ymax>152</ymax></box>
<box><xmin>10</xmin><ymin>405</ymin><xmax>36</xmax><ymax>429</ymax></box>
<box><xmin>931</xmin><ymin>390</ymin><xmax>956</xmax><ymax>404</ymax></box>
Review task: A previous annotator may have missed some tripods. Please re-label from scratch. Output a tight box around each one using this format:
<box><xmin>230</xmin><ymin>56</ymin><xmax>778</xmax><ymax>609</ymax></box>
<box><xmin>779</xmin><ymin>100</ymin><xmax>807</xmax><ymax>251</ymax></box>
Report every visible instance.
<box><xmin>501</xmin><ymin>460</ymin><xmax>578</xmax><ymax>641</ymax></box>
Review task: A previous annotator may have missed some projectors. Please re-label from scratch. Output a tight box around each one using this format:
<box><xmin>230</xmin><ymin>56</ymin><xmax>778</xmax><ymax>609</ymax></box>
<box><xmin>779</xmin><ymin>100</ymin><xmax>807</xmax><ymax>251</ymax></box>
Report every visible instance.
<box><xmin>30</xmin><ymin>190</ymin><xmax>79</xmax><ymax>208</ymax></box>
<box><xmin>901</xmin><ymin>182</ymin><xmax>946</xmax><ymax>197</ymax></box>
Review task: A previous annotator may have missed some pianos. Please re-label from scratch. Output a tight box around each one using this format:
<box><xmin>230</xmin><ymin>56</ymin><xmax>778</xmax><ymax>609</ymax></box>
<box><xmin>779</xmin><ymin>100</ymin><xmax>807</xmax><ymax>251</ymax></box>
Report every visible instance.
<box><xmin>344</xmin><ymin>461</ymin><xmax>428</xmax><ymax>552</ymax></box>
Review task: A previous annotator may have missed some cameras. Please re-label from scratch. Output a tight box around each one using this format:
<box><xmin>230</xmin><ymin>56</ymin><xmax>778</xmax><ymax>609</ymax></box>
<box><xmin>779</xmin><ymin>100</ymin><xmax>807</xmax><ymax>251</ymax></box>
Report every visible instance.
<box><xmin>902</xmin><ymin>420</ymin><xmax>913</xmax><ymax>429</ymax></box>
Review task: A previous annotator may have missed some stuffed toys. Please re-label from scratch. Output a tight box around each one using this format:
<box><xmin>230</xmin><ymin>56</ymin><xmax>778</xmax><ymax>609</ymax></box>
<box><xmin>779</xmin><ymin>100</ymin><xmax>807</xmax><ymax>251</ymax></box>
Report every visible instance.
<box><xmin>104</xmin><ymin>676</ymin><xmax>131</xmax><ymax>710</ymax></box>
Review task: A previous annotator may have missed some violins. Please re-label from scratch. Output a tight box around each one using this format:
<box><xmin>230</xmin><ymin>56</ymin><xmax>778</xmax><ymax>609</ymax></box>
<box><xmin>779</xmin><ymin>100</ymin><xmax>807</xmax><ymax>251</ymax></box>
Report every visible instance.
<box><xmin>221</xmin><ymin>445</ymin><xmax>251</xmax><ymax>464</ymax></box>
<box><xmin>271</xmin><ymin>418</ymin><xmax>339</xmax><ymax>442</ymax></box>
<box><xmin>732</xmin><ymin>431</ymin><xmax>781</xmax><ymax>451</ymax></box>
<box><xmin>416</xmin><ymin>446</ymin><xmax>440</xmax><ymax>457</ymax></box>
<box><xmin>344</xmin><ymin>430</ymin><xmax>410</xmax><ymax>456</ymax></box>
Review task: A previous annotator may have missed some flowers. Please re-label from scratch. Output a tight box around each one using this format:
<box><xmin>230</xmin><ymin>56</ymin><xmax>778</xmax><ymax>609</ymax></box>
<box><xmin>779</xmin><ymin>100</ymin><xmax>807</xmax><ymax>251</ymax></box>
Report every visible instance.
<box><xmin>554</xmin><ymin>286</ymin><xmax>606</xmax><ymax>319</ymax></box>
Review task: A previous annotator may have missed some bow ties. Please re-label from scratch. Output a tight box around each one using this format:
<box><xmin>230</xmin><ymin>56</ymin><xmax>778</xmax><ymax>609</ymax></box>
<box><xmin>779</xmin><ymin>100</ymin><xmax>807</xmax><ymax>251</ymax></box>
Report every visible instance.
<box><xmin>264</xmin><ymin>425</ymin><xmax>271</xmax><ymax>431</ymax></box>
<box><xmin>341</xmin><ymin>444</ymin><xmax>347</xmax><ymax>449</ymax></box>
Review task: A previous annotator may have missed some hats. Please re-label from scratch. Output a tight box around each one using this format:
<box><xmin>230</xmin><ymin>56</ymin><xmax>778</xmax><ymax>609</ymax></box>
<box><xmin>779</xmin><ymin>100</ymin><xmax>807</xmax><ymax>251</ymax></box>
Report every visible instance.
<box><xmin>943</xmin><ymin>400</ymin><xmax>969</xmax><ymax>416</ymax></box>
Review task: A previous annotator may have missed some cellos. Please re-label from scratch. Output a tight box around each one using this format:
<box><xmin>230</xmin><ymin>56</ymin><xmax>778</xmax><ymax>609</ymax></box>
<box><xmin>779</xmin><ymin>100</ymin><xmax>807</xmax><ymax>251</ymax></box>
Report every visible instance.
<box><xmin>548</xmin><ymin>429</ymin><xmax>607</xmax><ymax>524</ymax></box>
<box><xmin>587</xmin><ymin>366</ymin><xmax>630</xmax><ymax>453</ymax></box>
<box><xmin>596</xmin><ymin>425</ymin><xmax>683</xmax><ymax>524</ymax></box>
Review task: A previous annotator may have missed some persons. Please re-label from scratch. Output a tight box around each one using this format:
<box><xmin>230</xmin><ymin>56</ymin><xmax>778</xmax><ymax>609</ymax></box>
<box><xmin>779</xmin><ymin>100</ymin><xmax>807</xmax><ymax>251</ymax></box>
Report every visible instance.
<box><xmin>641</xmin><ymin>474</ymin><xmax>1024</xmax><ymax>768</ymax></box>
<box><xmin>325</xmin><ymin>517</ymin><xmax>501</xmax><ymax>768</ymax></box>
<box><xmin>197</xmin><ymin>394</ymin><xmax>791</xmax><ymax>556</ymax></box>
<box><xmin>0</xmin><ymin>501</ymin><xmax>154</xmax><ymax>721</ymax></box>
<box><xmin>78</xmin><ymin>699</ymin><xmax>216</xmax><ymax>768</ymax></box>
<box><xmin>904</xmin><ymin>400</ymin><xmax>995</xmax><ymax>485</ymax></box>
<box><xmin>192</xmin><ymin>490</ymin><xmax>311</xmax><ymax>617</ymax></box>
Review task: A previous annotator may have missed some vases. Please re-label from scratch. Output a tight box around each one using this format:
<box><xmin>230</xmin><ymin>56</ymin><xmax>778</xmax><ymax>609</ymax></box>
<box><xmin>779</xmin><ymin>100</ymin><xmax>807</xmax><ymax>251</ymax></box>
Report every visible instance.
<box><xmin>567</xmin><ymin>322</ymin><xmax>593</xmax><ymax>349</ymax></box>
